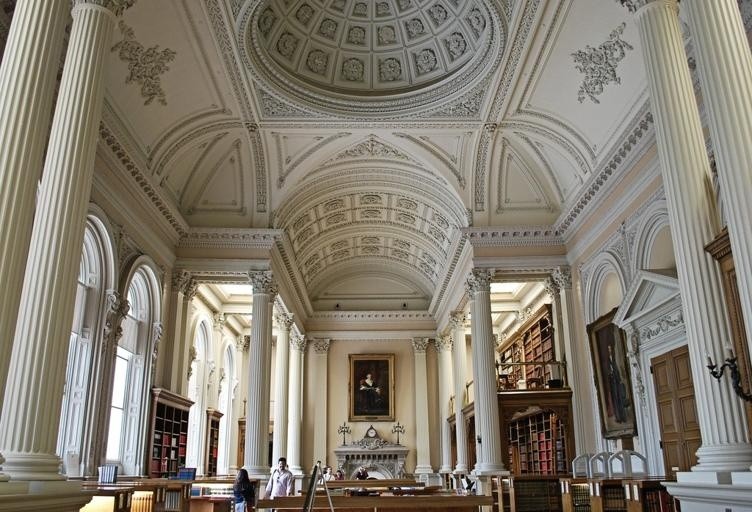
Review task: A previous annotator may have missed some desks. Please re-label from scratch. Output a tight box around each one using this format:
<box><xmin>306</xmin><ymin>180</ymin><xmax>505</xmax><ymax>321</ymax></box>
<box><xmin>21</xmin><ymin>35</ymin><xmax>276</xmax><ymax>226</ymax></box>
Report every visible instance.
<box><xmin>257</xmin><ymin>479</ymin><xmax>494</xmax><ymax>512</ymax></box>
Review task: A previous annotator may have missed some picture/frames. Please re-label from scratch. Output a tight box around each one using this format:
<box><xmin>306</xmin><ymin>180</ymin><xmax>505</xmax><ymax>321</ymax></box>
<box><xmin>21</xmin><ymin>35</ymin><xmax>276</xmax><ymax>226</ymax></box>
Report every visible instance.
<box><xmin>584</xmin><ymin>307</ymin><xmax>637</xmax><ymax>440</ymax></box>
<box><xmin>347</xmin><ymin>353</ymin><xmax>396</xmax><ymax>424</ymax></box>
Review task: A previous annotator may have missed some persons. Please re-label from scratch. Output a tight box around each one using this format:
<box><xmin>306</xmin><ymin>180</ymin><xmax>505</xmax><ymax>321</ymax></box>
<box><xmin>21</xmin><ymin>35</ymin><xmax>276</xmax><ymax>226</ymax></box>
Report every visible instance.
<box><xmin>317</xmin><ymin>466</ymin><xmax>335</xmax><ymax>486</ymax></box>
<box><xmin>263</xmin><ymin>457</ymin><xmax>294</xmax><ymax>512</ymax></box>
<box><xmin>234</xmin><ymin>469</ymin><xmax>256</xmax><ymax>512</ymax></box>
<box><xmin>604</xmin><ymin>344</ymin><xmax>627</xmax><ymax>424</ymax></box>
<box><xmin>357</xmin><ymin>467</ymin><xmax>368</xmax><ymax>481</ymax></box>
<box><xmin>334</xmin><ymin>471</ymin><xmax>345</xmax><ymax>481</ymax></box>
<box><xmin>360</xmin><ymin>373</ymin><xmax>385</xmax><ymax>408</ymax></box>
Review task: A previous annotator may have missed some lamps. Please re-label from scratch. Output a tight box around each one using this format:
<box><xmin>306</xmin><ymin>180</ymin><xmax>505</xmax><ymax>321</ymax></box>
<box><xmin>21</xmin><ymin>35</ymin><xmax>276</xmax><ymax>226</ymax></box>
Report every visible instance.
<box><xmin>703</xmin><ymin>340</ymin><xmax>752</xmax><ymax>405</ymax></box>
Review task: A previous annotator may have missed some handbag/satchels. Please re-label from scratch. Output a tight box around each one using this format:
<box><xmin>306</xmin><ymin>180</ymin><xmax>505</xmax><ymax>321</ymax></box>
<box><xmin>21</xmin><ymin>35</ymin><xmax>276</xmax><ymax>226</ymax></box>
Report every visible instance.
<box><xmin>244</xmin><ymin>500</ymin><xmax>255</xmax><ymax>512</ymax></box>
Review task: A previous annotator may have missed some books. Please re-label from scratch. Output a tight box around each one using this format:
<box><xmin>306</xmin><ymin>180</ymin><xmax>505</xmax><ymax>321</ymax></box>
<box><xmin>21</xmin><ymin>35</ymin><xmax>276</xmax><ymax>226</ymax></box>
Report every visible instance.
<box><xmin>168</xmin><ymin>465</ymin><xmax>196</xmax><ymax>480</ymax></box>
<box><xmin>129</xmin><ymin>481</ymin><xmax>233</xmax><ymax>512</ymax></box>
<box><xmin>149</xmin><ymin>431</ymin><xmax>188</xmax><ymax>477</ymax></box>
<box><xmin>503</xmin><ymin>318</ymin><xmax>550</xmax><ymax>384</ymax></box>
<box><xmin>96</xmin><ymin>463</ymin><xmax>118</xmax><ymax>485</ymax></box>
<box><xmin>507</xmin><ymin>416</ymin><xmax>565</xmax><ymax>474</ymax></box>
<box><xmin>491</xmin><ymin>476</ymin><xmax>681</xmax><ymax>512</ymax></box>
<box><xmin>213</xmin><ymin>431</ymin><xmax>219</xmax><ymax>456</ymax></box>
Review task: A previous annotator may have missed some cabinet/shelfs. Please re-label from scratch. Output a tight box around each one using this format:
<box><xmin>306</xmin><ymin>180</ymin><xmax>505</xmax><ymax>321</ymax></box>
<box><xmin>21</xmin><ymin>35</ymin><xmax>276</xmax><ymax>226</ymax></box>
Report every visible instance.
<box><xmin>206</xmin><ymin>409</ymin><xmax>225</xmax><ymax>478</ymax></box>
<box><xmin>485</xmin><ymin>474</ymin><xmax>680</xmax><ymax>512</ymax></box>
<box><xmin>493</xmin><ymin>304</ymin><xmax>553</xmax><ymax>390</ymax></box>
<box><xmin>79</xmin><ymin>477</ymin><xmax>260</xmax><ymax>512</ymax></box>
<box><xmin>148</xmin><ymin>388</ymin><xmax>194</xmax><ymax>478</ymax></box>
<box><xmin>506</xmin><ymin>410</ymin><xmax>567</xmax><ymax>479</ymax></box>
